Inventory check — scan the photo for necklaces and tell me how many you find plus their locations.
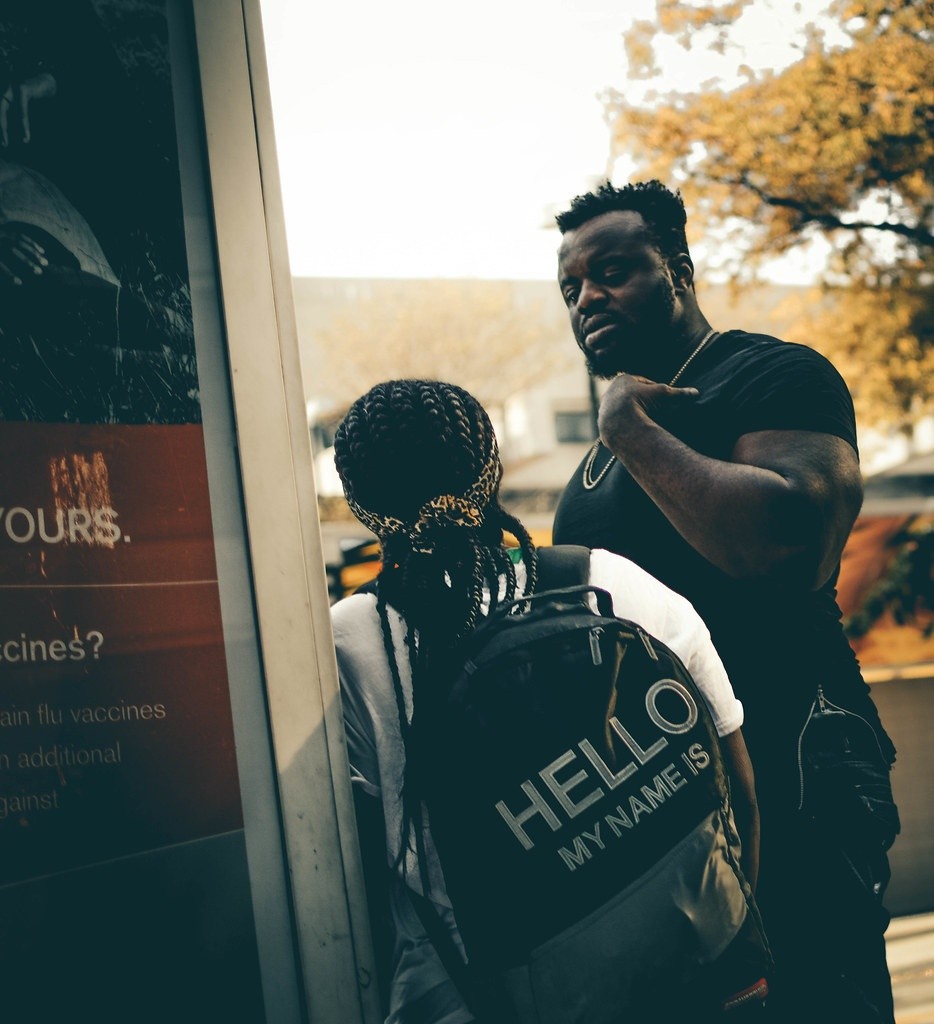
[582,329,721,490]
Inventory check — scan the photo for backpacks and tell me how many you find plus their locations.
[341,543,778,1023]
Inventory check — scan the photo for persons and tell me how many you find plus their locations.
[320,376,785,1024]
[538,179,902,1024]
